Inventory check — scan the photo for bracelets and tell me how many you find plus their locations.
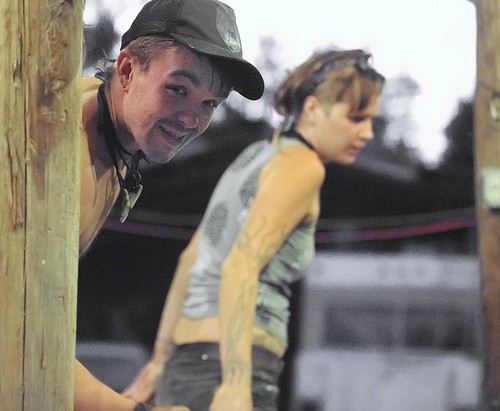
[133,402,152,410]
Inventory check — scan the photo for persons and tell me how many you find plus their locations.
[120,50,385,411]
[74,0,266,411]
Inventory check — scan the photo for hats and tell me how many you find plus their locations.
[120,0,265,100]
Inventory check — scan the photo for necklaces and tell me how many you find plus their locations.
[281,130,319,153]
[97,84,151,223]
